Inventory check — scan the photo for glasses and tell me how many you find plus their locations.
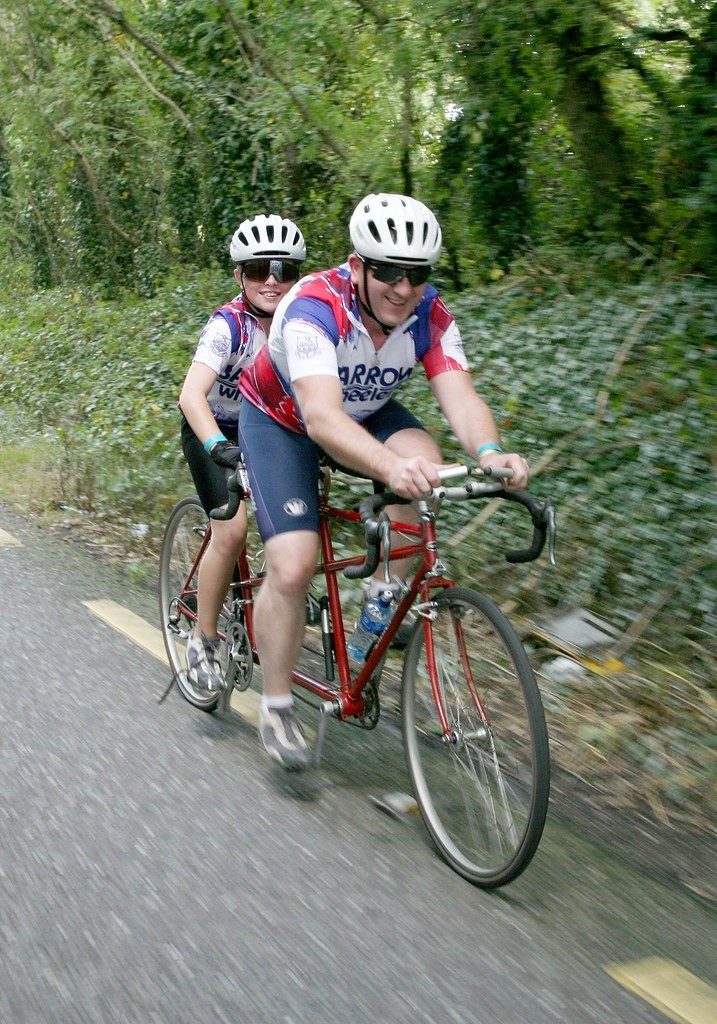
[355,252,431,287]
[241,260,299,284]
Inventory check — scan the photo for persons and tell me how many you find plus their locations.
[174,213,332,692]
[236,190,531,779]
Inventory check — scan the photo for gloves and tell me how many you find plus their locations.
[210,442,241,469]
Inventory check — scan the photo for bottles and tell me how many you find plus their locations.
[346,590,394,667]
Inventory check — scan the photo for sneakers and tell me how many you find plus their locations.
[258,702,315,772]
[359,585,422,646]
[183,629,225,693]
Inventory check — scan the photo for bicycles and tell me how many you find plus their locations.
[155,442,562,891]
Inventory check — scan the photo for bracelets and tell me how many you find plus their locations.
[477,442,505,456]
[478,448,502,468]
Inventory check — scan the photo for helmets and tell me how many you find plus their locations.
[348,194,442,267]
[230,213,306,263]
